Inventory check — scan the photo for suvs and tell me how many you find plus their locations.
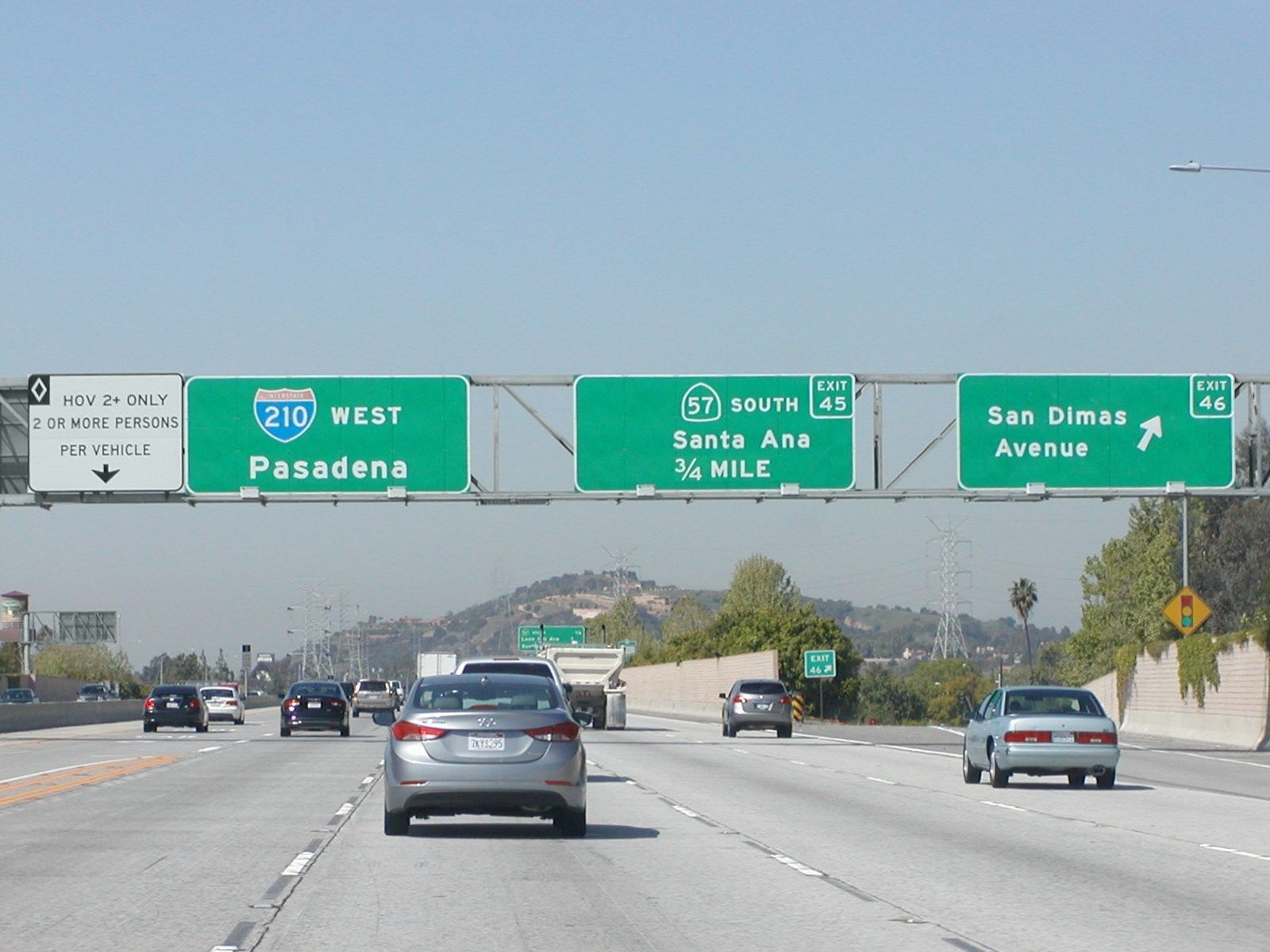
[719,680,792,739]
[350,679,395,719]
[452,657,593,729]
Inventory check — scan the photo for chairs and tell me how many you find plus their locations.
[296,689,309,695]
[511,694,538,709]
[432,695,463,710]
[324,688,335,695]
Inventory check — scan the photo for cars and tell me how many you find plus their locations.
[962,684,1123,791]
[275,681,352,739]
[371,674,590,842]
[77,684,115,702]
[0,688,39,704]
[142,686,210,735]
[200,687,246,724]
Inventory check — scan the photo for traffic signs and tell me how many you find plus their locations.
[803,650,837,678]
[572,375,856,494]
[517,626,585,652]
[956,369,1240,492]
[28,372,471,492]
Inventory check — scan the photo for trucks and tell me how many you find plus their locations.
[538,647,627,729]
